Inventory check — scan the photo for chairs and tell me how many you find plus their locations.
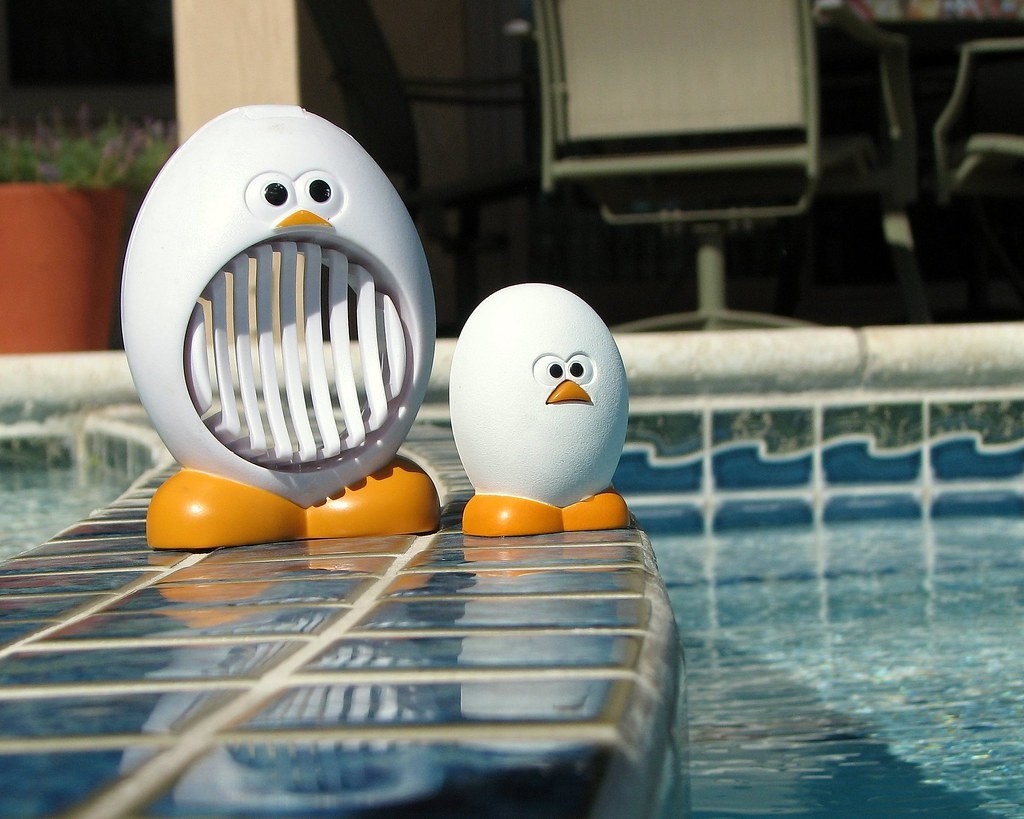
[531,1,1024,333]
[304,1,540,338]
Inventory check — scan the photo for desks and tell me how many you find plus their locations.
[814,19,1024,75]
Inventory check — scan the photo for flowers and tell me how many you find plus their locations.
[0,103,177,189]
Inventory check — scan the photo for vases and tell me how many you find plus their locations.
[0,181,125,353]
